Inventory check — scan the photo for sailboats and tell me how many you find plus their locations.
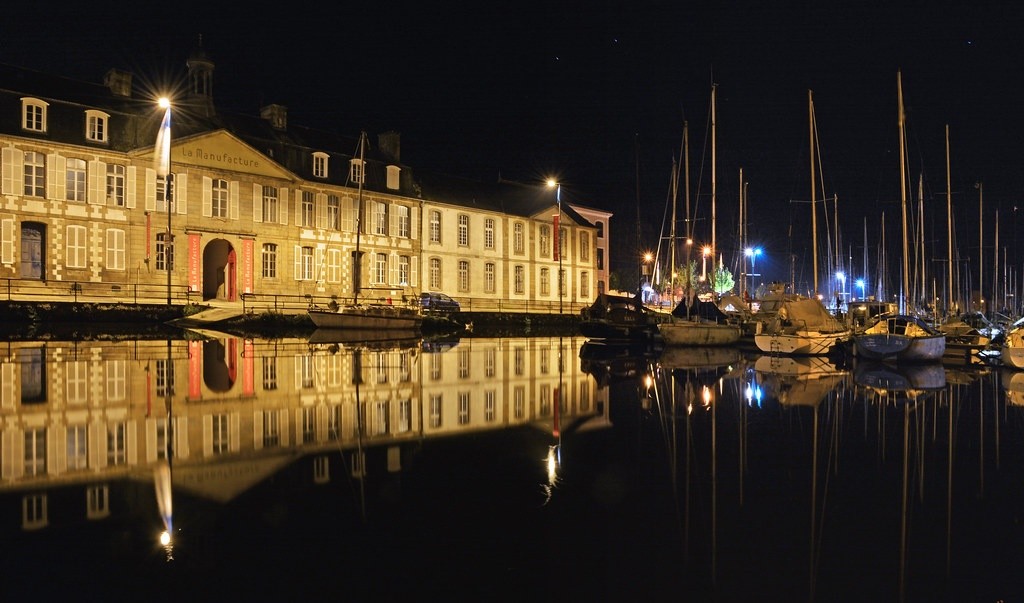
[659,71,1024,363]
[580,339,1024,602]
[308,328,422,522]
[306,131,458,342]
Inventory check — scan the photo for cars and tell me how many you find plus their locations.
[418,291,460,312]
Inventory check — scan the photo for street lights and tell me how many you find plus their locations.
[157,335,178,570]
[151,106,176,300]
[554,181,564,318]
[543,337,569,490]
